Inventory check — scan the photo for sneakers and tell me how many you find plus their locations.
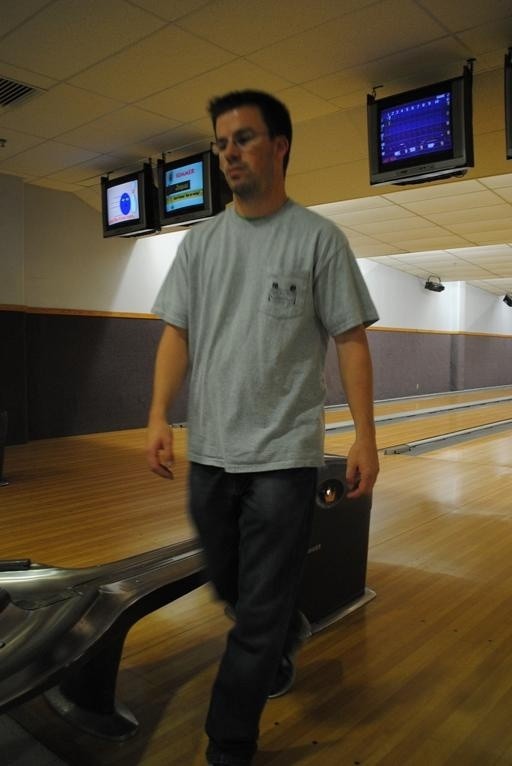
[268,611,311,699]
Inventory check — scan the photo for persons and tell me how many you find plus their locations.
[143,83,382,765]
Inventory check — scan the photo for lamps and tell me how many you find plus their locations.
[503,294,512,309]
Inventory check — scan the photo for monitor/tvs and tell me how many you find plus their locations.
[100,162,159,238]
[154,141,234,228]
[504,47,512,158]
[364,74,476,187]
